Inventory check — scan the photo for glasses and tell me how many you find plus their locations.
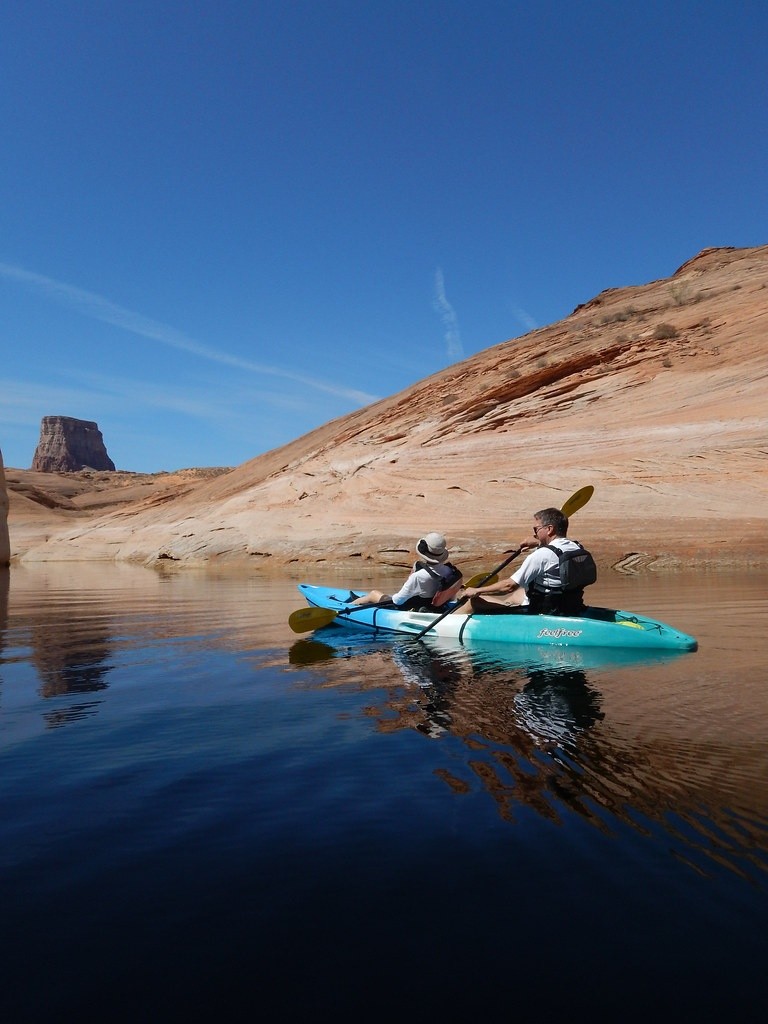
[533,524,550,536]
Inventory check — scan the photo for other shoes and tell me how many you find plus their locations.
[349,590,360,601]
[330,597,342,602]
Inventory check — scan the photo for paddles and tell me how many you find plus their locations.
[288,573,499,633]
[415,484,595,639]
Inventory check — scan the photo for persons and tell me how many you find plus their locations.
[452,507,583,618]
[329,532,455,612]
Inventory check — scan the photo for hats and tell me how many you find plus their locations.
[416,533,448,562]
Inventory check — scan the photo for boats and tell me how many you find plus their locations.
[297,584,698,650]
